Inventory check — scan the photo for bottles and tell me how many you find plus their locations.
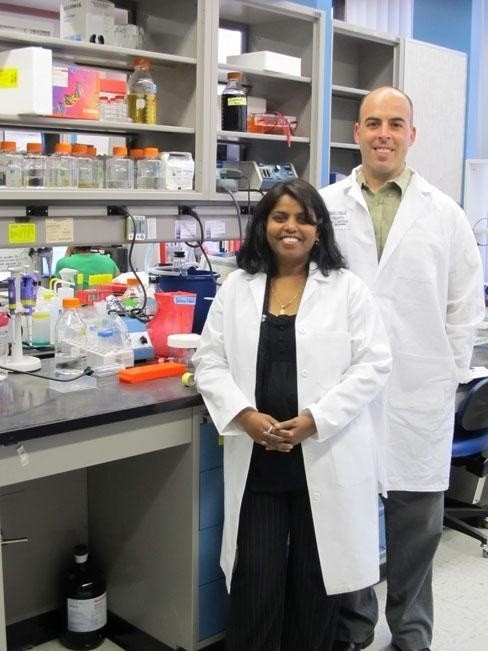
[0,140,162,189]
[32,312,51,346]
[123,57,157,127]
[76,273,116,300]
[54,298,86,375]
[0,313,10,382]
[59,545,109,651]
[121,280,146,317]
[172,252,187,277]
[221,72,247,134]
[88,327,112,355]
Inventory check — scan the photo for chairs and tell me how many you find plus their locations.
[442,379,488,557]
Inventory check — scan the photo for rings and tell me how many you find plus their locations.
[267,425,274,436]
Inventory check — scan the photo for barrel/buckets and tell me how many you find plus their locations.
[158,150,195,191]
[146,291,198,359]
[157,266,221,336]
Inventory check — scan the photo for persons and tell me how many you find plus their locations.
[55,246,121,290]
[316,87,485,651]
[193,180,390,651]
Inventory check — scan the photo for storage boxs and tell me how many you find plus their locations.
[227,48,302,79]
[114,8,146,50]
[49,60,129,121]
[1,46,53,116]
[57,1,116,46]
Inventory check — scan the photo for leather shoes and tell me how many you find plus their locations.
[331,631,373,650]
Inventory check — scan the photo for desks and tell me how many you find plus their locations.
[0,343,488,651]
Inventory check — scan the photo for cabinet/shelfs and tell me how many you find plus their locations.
[330,18,404,186]
[213,0,326,203]
[0,0,212,208]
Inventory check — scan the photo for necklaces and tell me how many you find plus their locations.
[267,275,305,317]
